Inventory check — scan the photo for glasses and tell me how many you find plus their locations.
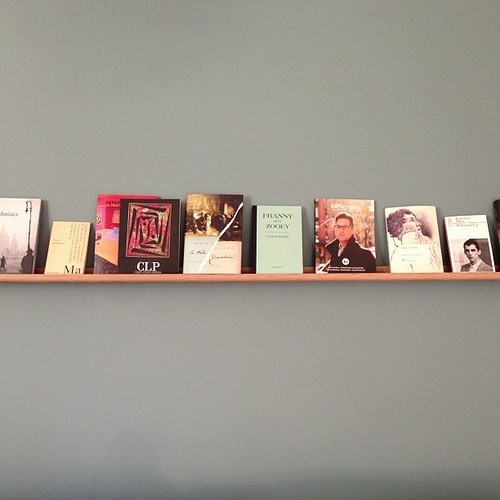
[334,224,351,230]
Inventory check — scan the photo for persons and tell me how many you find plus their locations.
[386,208,441,271]
[460,239,493,271]
[323,211,376,273]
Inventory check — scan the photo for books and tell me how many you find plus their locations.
[44,220,91,275]
[118,197,181,274]
[250,204,304,274]
[443,214,496,272]
[182,193,244,274]
[313,198,376,273]
[384,205,445,274]
[0,197,42,275]
[92,194,159,274]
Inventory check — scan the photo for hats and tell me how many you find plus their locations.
[26,249,34,252]
